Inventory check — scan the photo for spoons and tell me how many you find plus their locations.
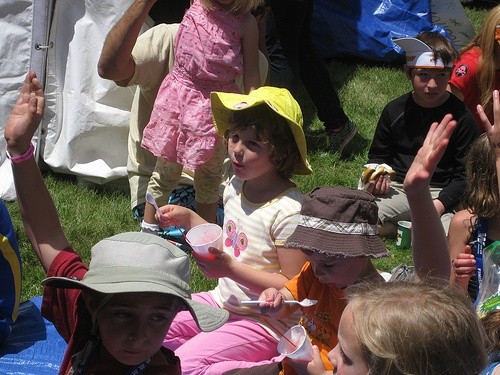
[240,298,318,307]
[146,192,168,221]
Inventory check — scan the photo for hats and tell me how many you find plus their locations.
[40,232,230,331]
[283,186,391,259]
[391,36,454,70]
[209,85,312,176]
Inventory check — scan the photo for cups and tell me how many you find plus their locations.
[276,325,314,362]
[395,220,411,250]
[185,223,223,262]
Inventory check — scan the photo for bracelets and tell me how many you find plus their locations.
[5,142,34,163]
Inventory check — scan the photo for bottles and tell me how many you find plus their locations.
[467,218,488,299]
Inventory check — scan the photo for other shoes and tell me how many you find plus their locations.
[326,122,368,164]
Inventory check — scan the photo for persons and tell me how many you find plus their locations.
[265,0,358,156]
[449,132,500,320]
[140,0,263,235]
[365,31,477,238]
[155,87,313,375]
[259,186,392,375]
[446,4,500,133]
[0,198,22,348]
[4,71,229,375]
[327,115,489,375]
[97,0,268,250]
